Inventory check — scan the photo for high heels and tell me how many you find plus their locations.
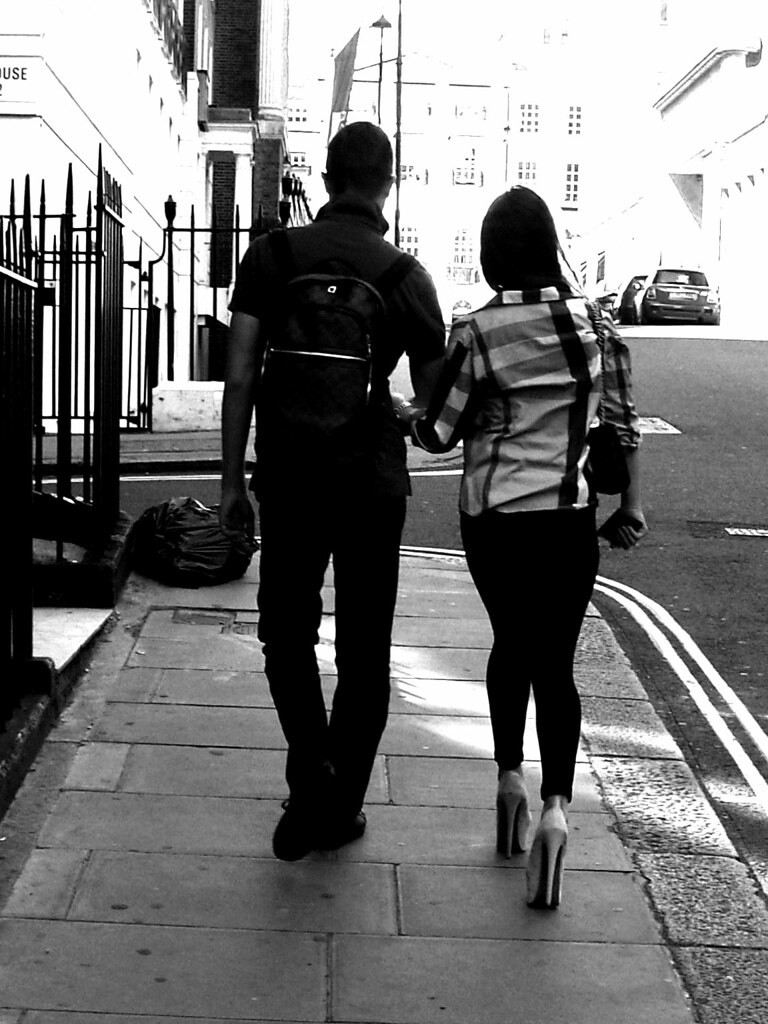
[496,772,529,860]
[524,808,569,910]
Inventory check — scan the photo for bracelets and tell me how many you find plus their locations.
[394,401,412,418]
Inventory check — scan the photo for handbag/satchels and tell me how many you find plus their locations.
[581,302,632,494]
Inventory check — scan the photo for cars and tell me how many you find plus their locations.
[619,269,720,325]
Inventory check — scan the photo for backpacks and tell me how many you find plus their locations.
[258,228,407,431]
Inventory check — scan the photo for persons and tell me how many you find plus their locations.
[217,123,448,861]
[388,184,647,908]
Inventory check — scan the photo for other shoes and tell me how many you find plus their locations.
[316,811,366,854]
[272,751,338,861]
